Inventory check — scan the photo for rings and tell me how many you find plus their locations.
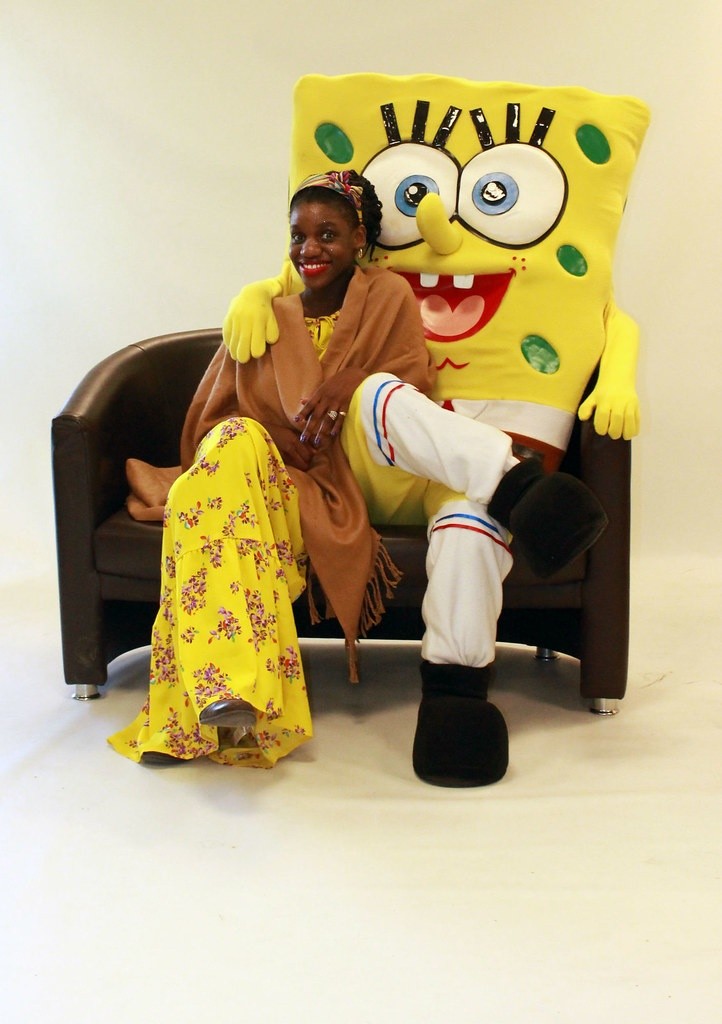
[339,411,346,416]
[326,411,338,420]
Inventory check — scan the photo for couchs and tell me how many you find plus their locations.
[51,328,632,715]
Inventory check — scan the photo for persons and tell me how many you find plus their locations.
[105,169,436,770]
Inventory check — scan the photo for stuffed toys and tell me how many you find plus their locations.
[221,73,651,789]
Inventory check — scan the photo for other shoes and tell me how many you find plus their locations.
[143,751,185,765]
[199,699,258,726]
